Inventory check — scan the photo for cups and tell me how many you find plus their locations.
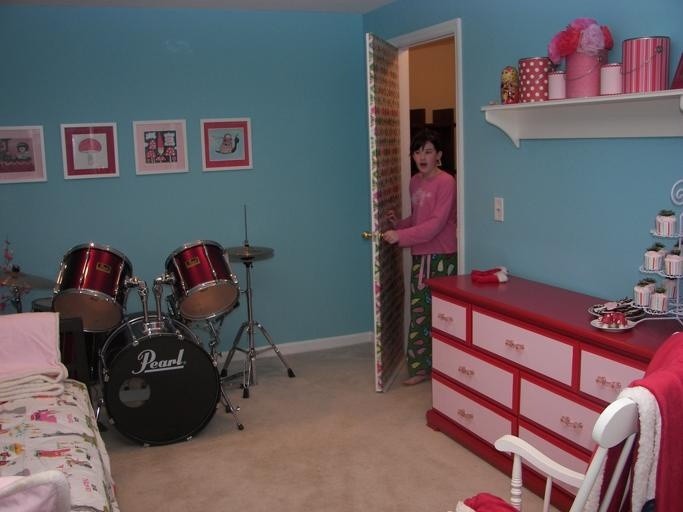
[548,70,567,101]
[599,62,623,97]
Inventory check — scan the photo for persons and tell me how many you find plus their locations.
[384,130,457,385]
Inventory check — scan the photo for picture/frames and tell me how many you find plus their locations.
[0,126,47,184]
[60,121,120,180]
[200,118,254,172]
[133,118,189,175]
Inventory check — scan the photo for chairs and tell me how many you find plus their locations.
[454,332,683,511]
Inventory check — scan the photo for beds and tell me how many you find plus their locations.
[0,366,113,512]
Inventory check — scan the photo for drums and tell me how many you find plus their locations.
[166,239,238,321]
[100,315,222,445]
[33,297,53,310]
[53,241,131,332]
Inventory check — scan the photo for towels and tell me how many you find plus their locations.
[584,385,662,512]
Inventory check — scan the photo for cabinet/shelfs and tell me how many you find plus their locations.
[423,272,682,511]
[589,179,683,333]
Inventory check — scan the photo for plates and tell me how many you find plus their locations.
[587,303,647,320]
[590,318,636,333]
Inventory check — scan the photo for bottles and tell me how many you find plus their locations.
[501,65,520,104]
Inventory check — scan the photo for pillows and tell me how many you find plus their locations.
[0,469,71,512]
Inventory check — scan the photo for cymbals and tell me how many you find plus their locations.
[226,246,273,261]
[2,270,57,290]
